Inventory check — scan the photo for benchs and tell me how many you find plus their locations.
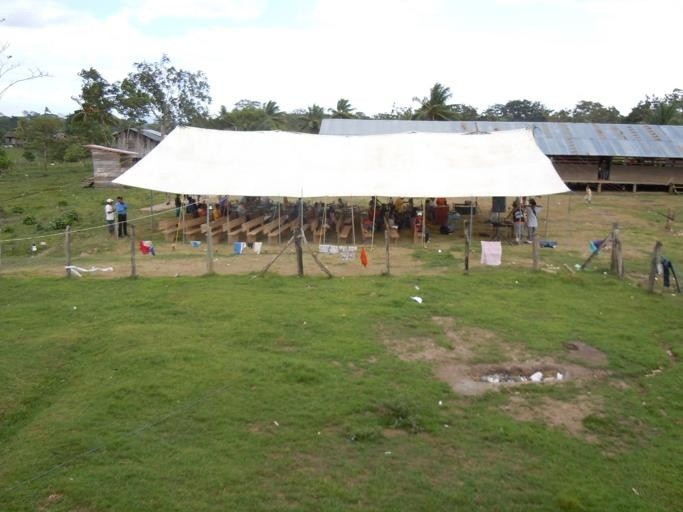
[157,210,423,245]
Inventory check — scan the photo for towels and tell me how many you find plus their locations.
[481,241,502,267]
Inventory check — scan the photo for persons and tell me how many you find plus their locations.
[114,196,128,238]
[584,184,592,206]
[174,194,461,237]
[504,195,543,243]
[104,198,116,233]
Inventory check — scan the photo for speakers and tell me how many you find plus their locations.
[492,197,506,213]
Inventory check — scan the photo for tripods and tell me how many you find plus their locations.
[489,212,513,245]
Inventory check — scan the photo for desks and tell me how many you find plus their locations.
[455,204,476,215]
[433,206,448,224]
